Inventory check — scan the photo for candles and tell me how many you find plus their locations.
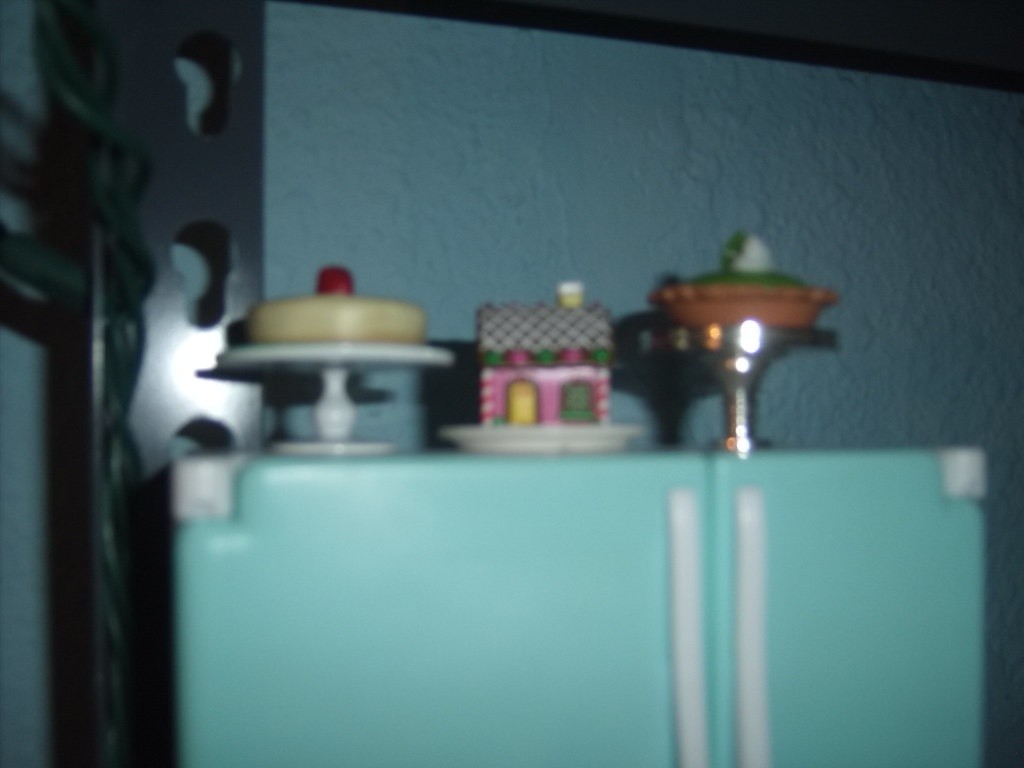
[669,488,713,768]
[737,488,774,768]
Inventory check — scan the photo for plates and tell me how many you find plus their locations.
[433,417,646,458]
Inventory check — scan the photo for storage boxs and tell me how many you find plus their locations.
[175,449,987,768]
